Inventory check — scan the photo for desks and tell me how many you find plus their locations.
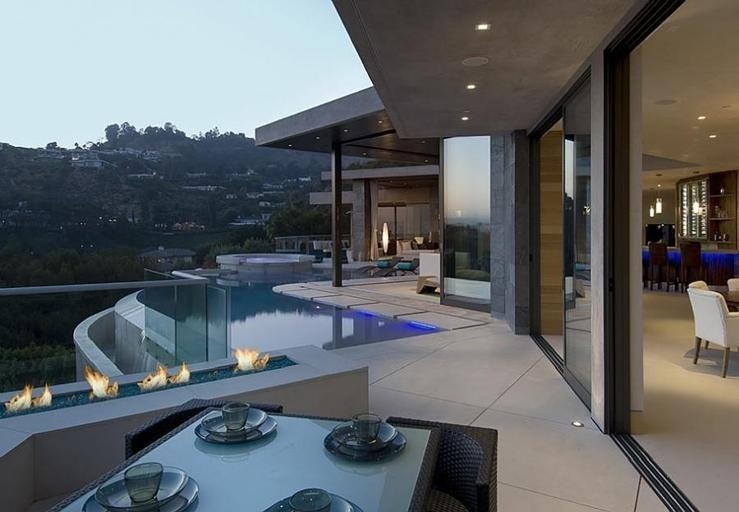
[642,248,739,286]
[45,406,445,511]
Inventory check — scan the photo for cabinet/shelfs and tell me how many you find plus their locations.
[707,169,739,243]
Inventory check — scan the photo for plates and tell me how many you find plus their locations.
[324,419,407,462]
[262,491,364,512]
[194,409,278,444]
[83,465,199,512]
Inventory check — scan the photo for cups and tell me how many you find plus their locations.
[352,411,381,445]
[124,462,163,505]
[221,401,250,432]
[288,488,333,512]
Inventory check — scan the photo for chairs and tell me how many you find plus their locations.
[685,277,739,377]
[648,242,679,292]
[386,412,499,511]
[680,241,704,295]
[122,396,284,458]
[306,236,440,293]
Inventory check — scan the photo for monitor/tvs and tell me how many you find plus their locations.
[646,224,676,246]
